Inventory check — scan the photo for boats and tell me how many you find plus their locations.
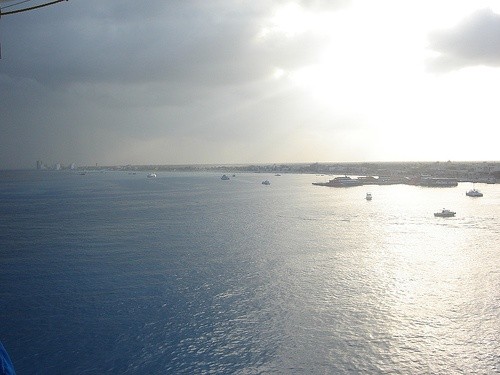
[465,189,484,198]
[426,174,498,189]
[221,174,229,181]
[432,207,456,217]
[147,173,157,178]
[366,193,373,200]
[261,180,270,184]
